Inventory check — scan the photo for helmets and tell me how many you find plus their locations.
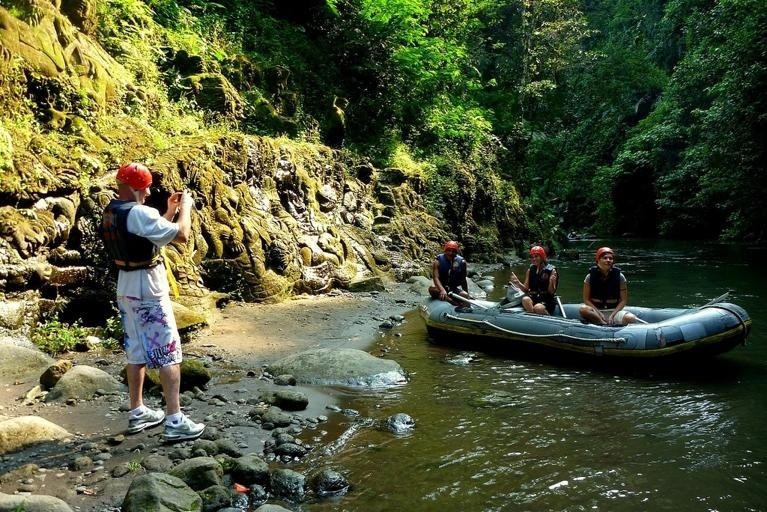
[596,247,614,261]
[445,241,458,251]
[531,246,546,260]
[116,163,153,191]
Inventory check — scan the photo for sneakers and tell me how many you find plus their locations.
[128,407,165,434]
[162,414,205,440]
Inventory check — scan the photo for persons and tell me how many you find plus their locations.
[102,162,207,442]
[427,240,477,306]
[509,245,559,316]
[567,229,575,239]
[578,247,641,326]
[568,232,580,240]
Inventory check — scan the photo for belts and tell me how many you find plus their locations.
[115,254,159,267]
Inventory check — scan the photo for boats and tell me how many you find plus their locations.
[414,293,754,369]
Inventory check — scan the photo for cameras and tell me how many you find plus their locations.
[177,193,183,202]
